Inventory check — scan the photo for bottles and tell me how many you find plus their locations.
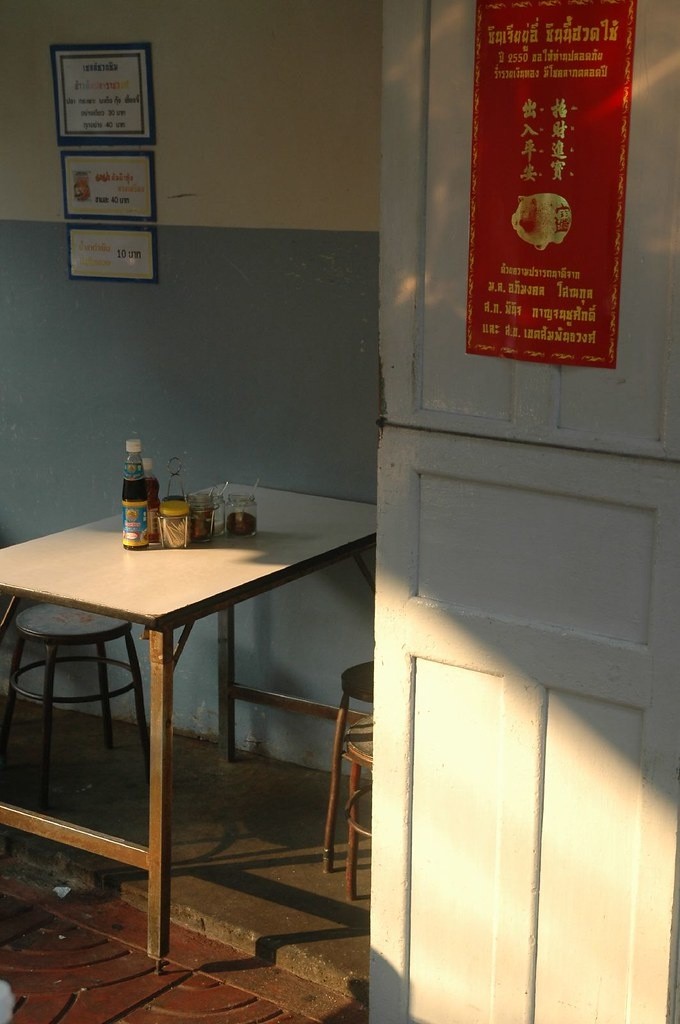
[225,493,257,538]
[158,500,191,548]
[141,458,161,545]
[211,493,226,537]
[186,493,215,542]
[121,438,150,550]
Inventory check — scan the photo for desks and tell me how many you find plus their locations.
[0,483,372,975]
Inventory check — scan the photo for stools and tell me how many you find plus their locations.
[323,660,373,900]
[0,603,149,811]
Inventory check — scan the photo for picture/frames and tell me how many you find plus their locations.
[49,43,158,148]
[66,223,159,284]
[60,150,158,223]
